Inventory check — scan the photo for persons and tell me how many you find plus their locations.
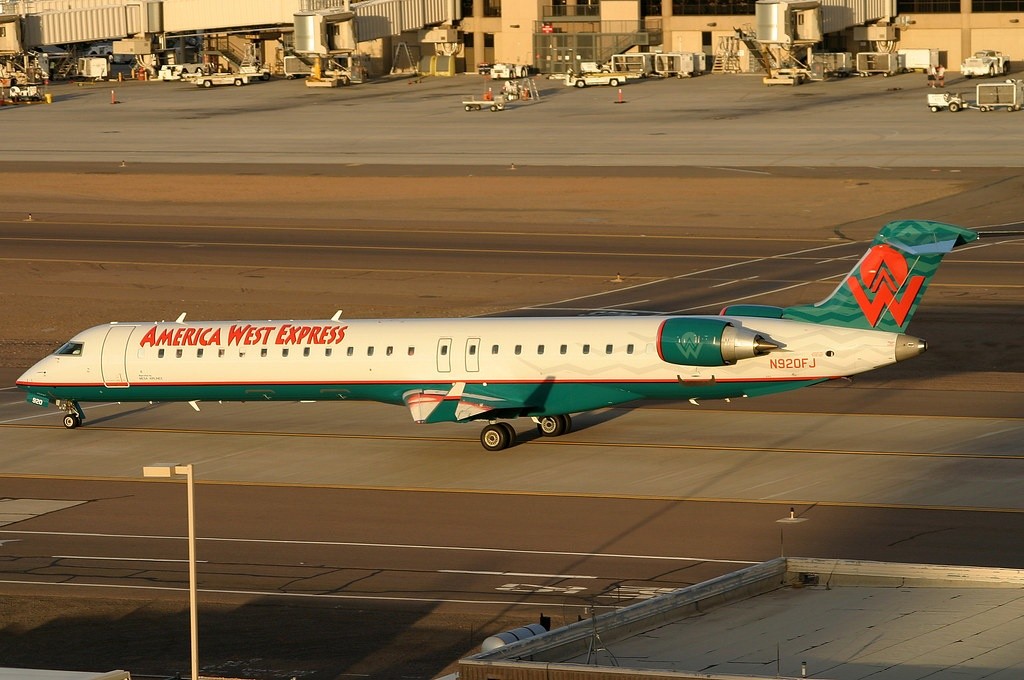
[926,63,946,88]
[49,60,56,81]
[72,346,80,355]
[568,68,586,82]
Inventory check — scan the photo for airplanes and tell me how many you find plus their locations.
[13,212,977,452]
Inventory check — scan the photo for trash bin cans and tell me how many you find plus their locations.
[478,63,490,75]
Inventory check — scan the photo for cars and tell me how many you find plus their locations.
[832,67,855,79]
[961,49,1011,80]
